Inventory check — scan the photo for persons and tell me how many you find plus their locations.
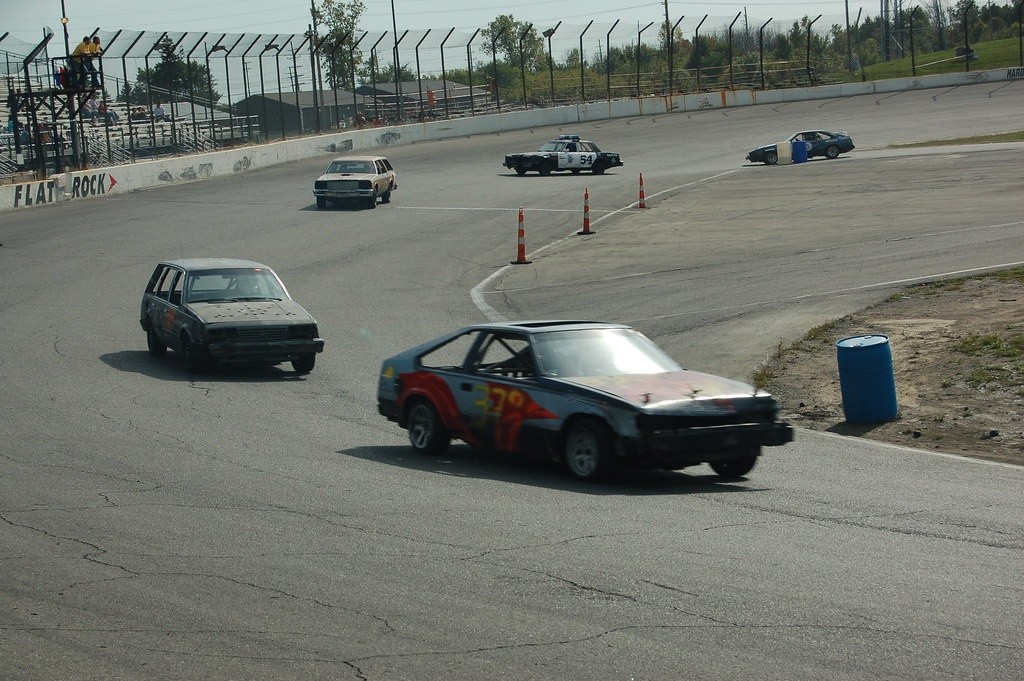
[7,113,54,143]
[67,35,104,89]
[132,101,170,122]
[82,90,120,127]
[228,270,262,295]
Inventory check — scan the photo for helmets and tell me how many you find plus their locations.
[236,273,259,293]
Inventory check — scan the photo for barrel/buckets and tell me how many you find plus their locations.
[793,141,807,162]
[836,334,898,423]
[777,142,792,164]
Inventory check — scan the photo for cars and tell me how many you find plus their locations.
[746,130,855,164]
[503,134,624,176]
[378,320,794,482]
[138,259,324,374]
[313,156,397,209]
